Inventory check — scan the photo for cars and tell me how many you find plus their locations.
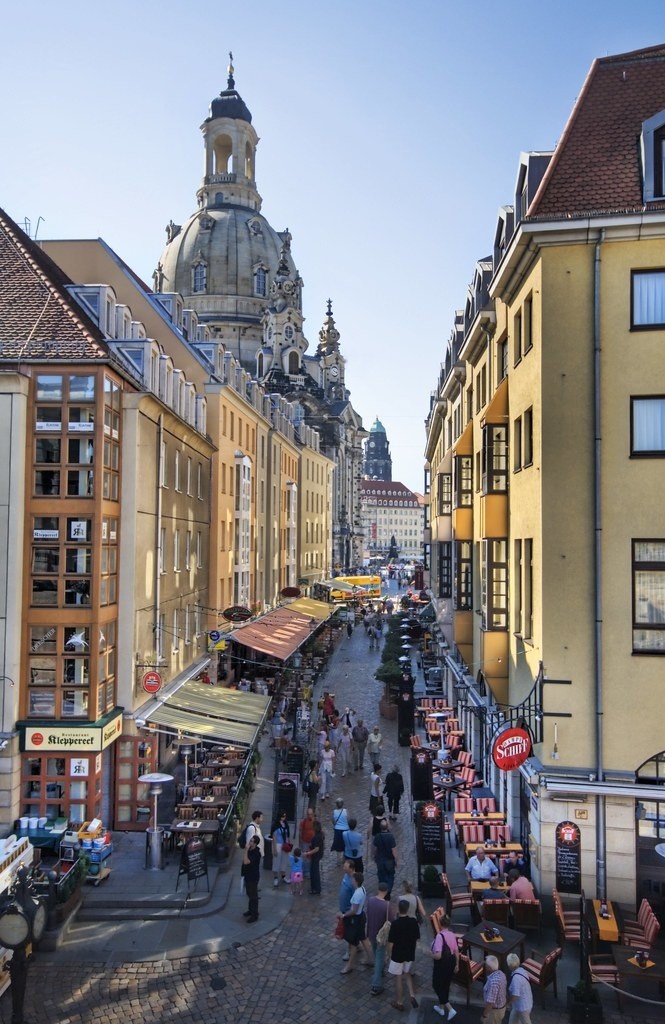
[332,612,363,623]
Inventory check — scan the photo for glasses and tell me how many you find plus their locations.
[281,815,286,818]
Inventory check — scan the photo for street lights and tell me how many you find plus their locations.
[1,864,50,1024]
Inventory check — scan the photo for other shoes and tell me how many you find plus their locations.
[389,815,397,822]
[308,889,320,894]
[340,966,353,974]
[410,996,418,1009]
[354,766,363,771]
[342,769,351,777]
[342,952,350,961]
[321,792,329,800]
[243,911,254,917]
[391,1000,404,1011]
[246,916,258,923]
[356,947,361,952]
[359,959,375,967]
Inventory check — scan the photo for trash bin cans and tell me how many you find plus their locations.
[408,608,415,617]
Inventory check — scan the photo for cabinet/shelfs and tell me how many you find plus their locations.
[59,839,112,886]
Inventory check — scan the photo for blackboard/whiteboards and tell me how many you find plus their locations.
[416,821,446,865]
[277,788,297,824]
[287,754,304,784]
[556,843,580,886]
[186,849,208,879]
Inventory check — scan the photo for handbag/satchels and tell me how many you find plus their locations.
[376,920,391,946]
[282,842,293,852]
[335,918,344,940]
[431,933,451,960]
[368,628,371,635]
[302,770,320,794]
[415,909,424,925]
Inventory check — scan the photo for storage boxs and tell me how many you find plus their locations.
[91,843,114,862]
[54,818,68,829]
[93,836,106,849]
[44,821,54,830]
[78,822,103,839]
[81,838,92,847]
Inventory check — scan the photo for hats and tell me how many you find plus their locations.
[508,869,519,877]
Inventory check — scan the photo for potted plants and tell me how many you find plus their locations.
[373,660,404,720]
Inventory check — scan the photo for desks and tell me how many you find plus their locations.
[585,899,625,947]
[468,880,536,899]
[453,812,505,825]
[168,746,247,855]
[421,742,452,759]
[432,758,465,774]
[8,828,71,854]
[471,788,496,799]
[460,920,526,972]
[464,841,524,863]
[433,774,467,812]
[612,945,665,1009]
[427,712,451,723]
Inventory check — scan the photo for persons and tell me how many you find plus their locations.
[240,557,536,1024]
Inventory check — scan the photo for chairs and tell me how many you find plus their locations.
[279,626,343,705]
[177,804,193,816]
[180,808,194,819]
[188,787,202,796]
[222,768,236,776]
[203,808,217,821]
[212,786,229,795]
[198,768,214,776]
[410,697,661,1010]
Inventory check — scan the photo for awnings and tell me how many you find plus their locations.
[320,577,364,596]
[226,607,325,662]
[280,596,341,621]
[139,680,272,749]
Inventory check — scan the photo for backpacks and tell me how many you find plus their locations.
[237,824,256,849]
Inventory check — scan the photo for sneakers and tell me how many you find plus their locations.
[274,879,278,887]
[282,877,291,884]
[434,1006,444,1015]
[447,1008,457,1020]
[370,986,384,995]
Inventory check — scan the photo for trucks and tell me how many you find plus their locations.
[331,575,381,601]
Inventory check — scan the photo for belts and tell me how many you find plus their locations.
[492,1005,505,1009]
